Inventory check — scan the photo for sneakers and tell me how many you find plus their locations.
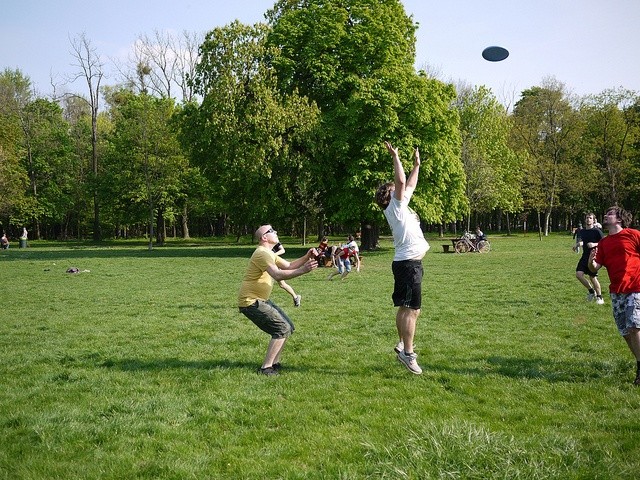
[272,363,282,369]
[588,290,597,301]
[394,342,416,354]
[398,350,422,374]
[596,297,604,304]
[294,295,301,307]
[258,367,278,375]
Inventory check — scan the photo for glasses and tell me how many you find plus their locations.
[350,248,354,250]
[260,228,273,239]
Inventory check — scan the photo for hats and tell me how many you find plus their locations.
[349,244,355,247]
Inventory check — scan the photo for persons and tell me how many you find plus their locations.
[316,237,330,268]
[1,233,10,250]
[238,224,319,376]
[374,141,431,376]
[594,217,603,230]
[328,245,360,281]
[345,235,360,267]
[460,230,476,240]
[272,242,301,307]
[572,223,582,253]
[20,227,28,240]
[474,227,487,251]
[573,211,605,304]
[588,204,640,386]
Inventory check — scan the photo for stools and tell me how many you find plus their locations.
[442,245,450,253]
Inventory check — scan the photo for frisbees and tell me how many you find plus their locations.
[482,46,509,62]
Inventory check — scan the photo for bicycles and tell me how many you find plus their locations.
[454,233,490,254]
